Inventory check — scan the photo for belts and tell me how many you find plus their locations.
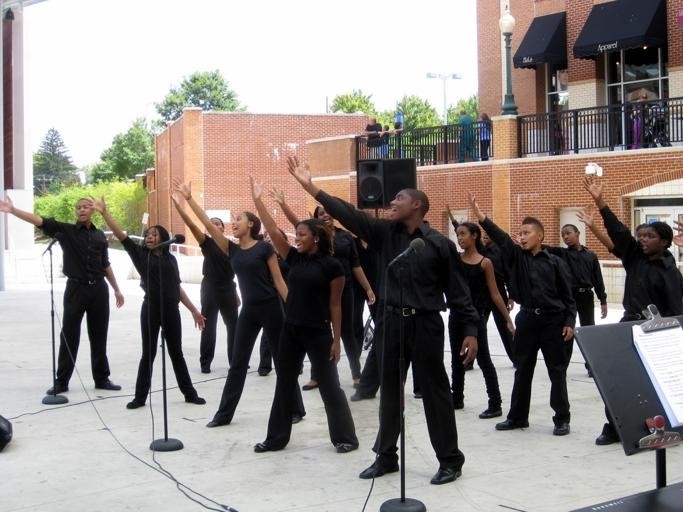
[523,306,559,315]
[384,304,423,317]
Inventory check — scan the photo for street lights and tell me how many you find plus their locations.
[498,3,520,115]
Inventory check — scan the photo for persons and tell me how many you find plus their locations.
[466,188,575,436]
[576,176,683,444]
[366,117,402,159]
[0,189,127,396]
[268,185,423,400]
[284,154,482,485]
[477,113,491,161]
[249,175,359,453]
[446,205,514,418]
[455,110,480,163]
[166,175,289,425]
[84,193,210,410]
[512,224,607,377]
[631,93,671,149]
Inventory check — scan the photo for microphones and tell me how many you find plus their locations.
[151,234,185,251]
[386,238,426,270]
[43,232,64,255]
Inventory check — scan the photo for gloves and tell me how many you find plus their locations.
[573,287,592,294]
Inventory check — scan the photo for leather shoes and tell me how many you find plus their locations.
[47,363,621,484]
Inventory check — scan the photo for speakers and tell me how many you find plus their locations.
[356,157,417,210]
